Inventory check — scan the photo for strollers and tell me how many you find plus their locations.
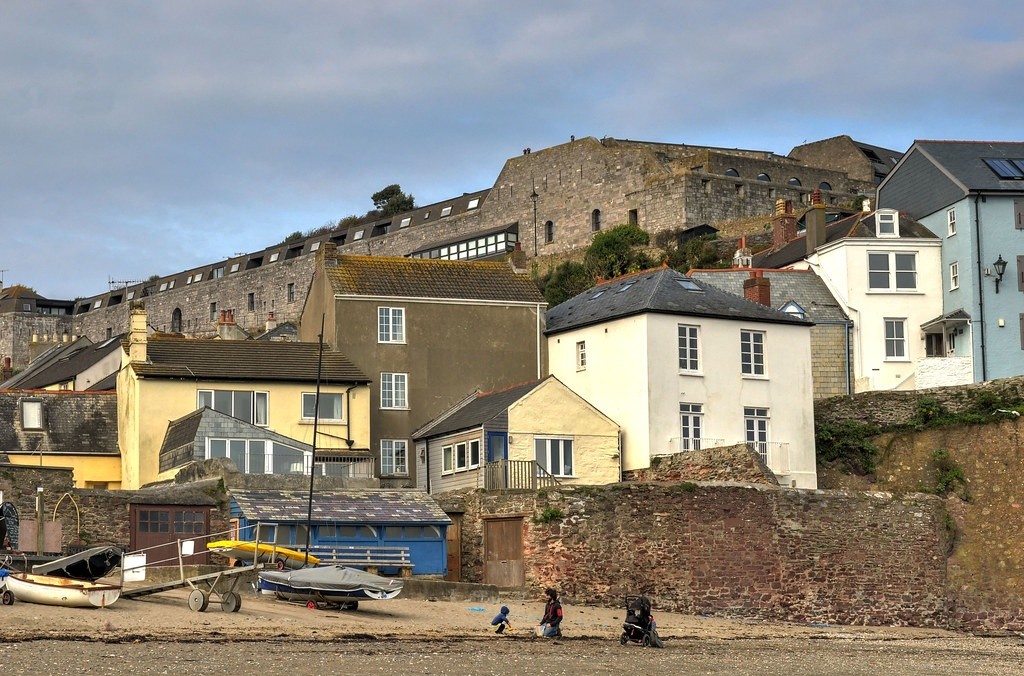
[621,594,658,646]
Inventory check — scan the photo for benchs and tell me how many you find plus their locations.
[276,544,415,579]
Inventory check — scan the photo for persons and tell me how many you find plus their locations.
[537,588,563,636]
[491,605,511,633]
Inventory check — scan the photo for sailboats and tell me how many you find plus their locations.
[253,312,405,603]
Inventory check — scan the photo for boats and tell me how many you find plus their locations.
[33,546,123,581]
[208,540,320,570]
[4,572,122,608]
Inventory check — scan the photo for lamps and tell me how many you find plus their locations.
[993,254,1008,293]
